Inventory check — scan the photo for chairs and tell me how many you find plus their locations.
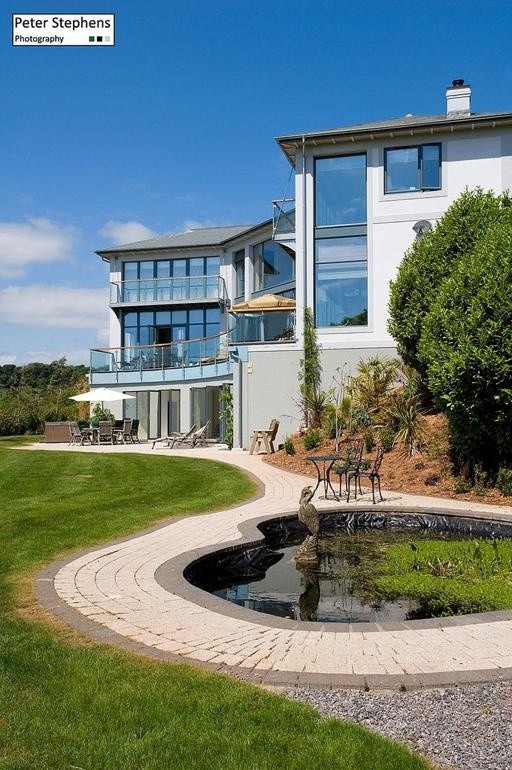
[328,441,386,503]
[151,418,212,450]
[249,419,280,456]
[67,418,141,446]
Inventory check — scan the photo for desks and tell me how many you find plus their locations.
[304,455,340,502]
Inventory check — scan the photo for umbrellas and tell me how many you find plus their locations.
[68,385,137,421]
[229,292,295,315]
